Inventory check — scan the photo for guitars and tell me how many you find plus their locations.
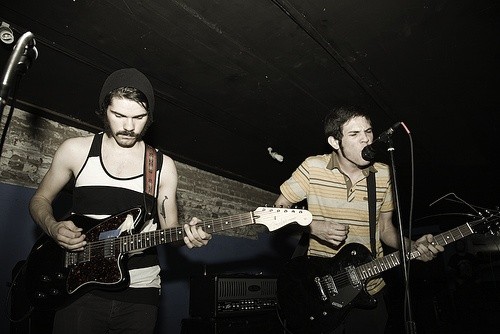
[24,203,313,311]
[276,205,500,334]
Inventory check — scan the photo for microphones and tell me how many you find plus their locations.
[361,122,403,161]
[0,31,38,120]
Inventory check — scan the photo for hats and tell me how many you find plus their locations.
[98,68,154,114]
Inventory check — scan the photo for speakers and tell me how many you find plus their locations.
[181,315,280,334]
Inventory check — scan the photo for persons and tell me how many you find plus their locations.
[29,68,211,334]
[272,103,445,334]
